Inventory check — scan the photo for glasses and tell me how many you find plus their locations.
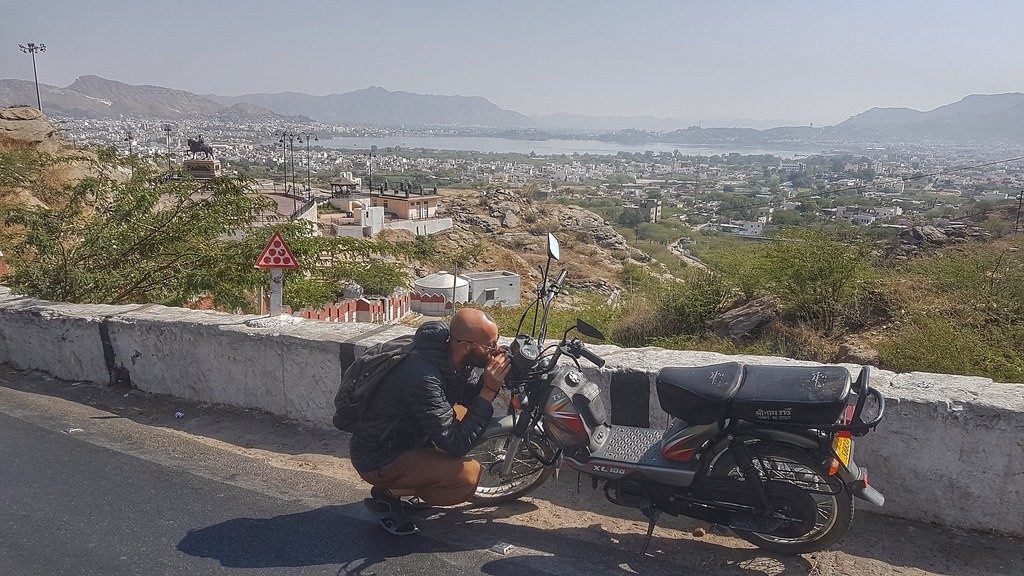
[457,339,497,354]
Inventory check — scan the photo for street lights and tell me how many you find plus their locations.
[274,129,293,193]
[363,149,376,195]
[124,130,134,175]
[298,132,319,200]
[280,134,303,213]
[18,42,47,111]
[163,123,173,170]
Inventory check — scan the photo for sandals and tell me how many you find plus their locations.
[364,486,418,535]
[399,493,431,510]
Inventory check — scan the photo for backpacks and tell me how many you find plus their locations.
[334,334,449,420]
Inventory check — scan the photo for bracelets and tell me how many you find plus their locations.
[483,384,496,393]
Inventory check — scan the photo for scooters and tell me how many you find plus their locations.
[461,232,887,560]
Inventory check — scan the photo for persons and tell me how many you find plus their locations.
[197,134,204,146]
[349,308,511,535]
